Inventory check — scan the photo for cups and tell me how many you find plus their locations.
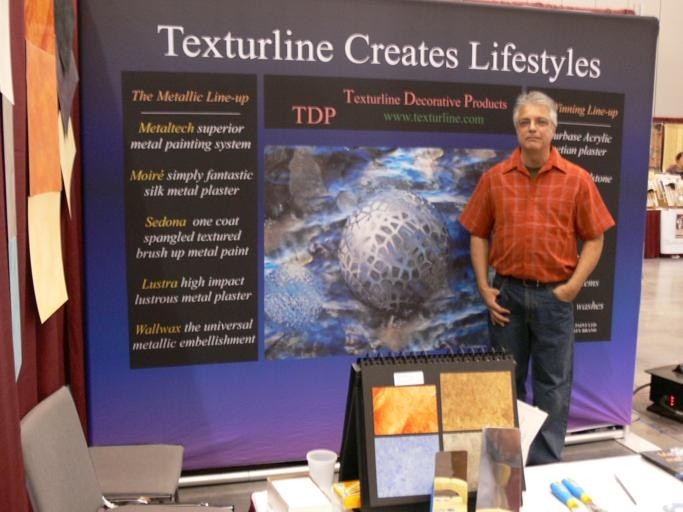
[306,448,338,492]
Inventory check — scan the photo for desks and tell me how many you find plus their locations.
[248,457,683,512]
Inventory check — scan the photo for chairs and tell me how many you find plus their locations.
[20,385,234,512]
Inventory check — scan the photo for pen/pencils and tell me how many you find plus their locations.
[613,472,638,506]
[550,480,607,512]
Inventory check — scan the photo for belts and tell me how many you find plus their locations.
[513,278,567,289]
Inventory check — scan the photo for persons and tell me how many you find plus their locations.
[456,91,618,468]
[666,154,682,178]
